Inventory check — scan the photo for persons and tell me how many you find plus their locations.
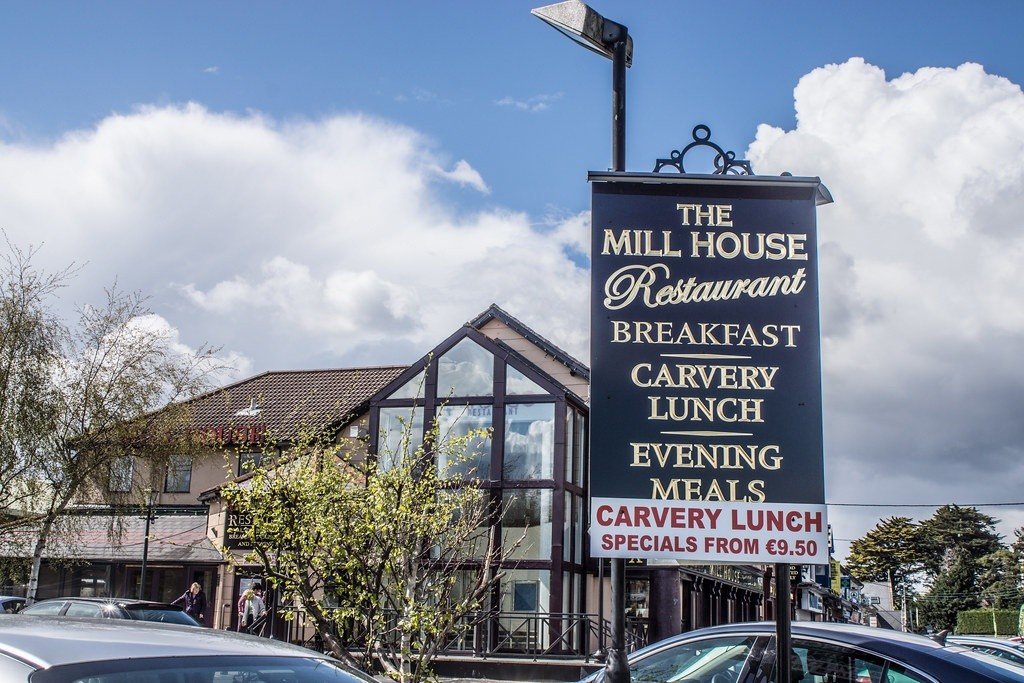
[171,582,207,623]
[236,582,268,633]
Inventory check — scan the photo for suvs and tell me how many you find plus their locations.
[15,596,202,627]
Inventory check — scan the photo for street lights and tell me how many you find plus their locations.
[528,0,636,683]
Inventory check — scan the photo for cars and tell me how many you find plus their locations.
[0,596,39,614]
[577,622,1024,683]
[0,614,383,683]
